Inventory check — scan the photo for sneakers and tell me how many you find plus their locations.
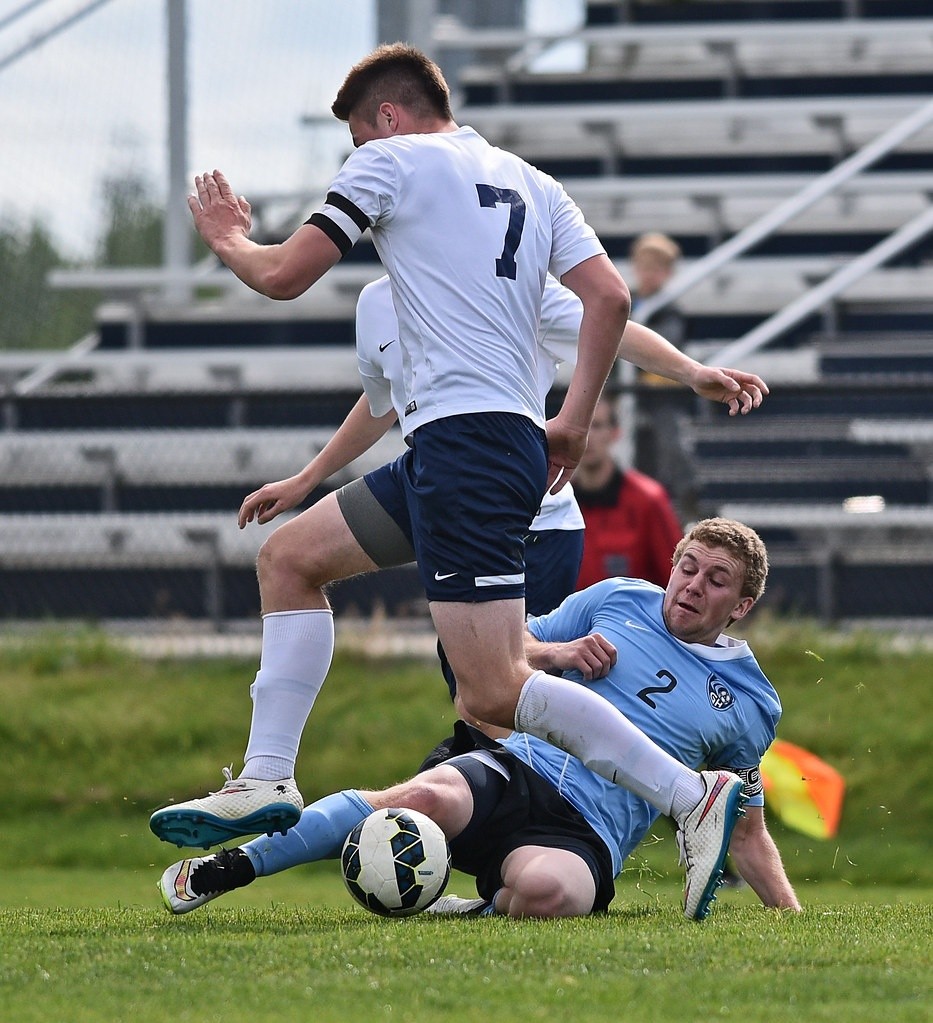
[156,847,243,913]
[674,768,752,920]
[147,762,304,850]
[426,893,488,916]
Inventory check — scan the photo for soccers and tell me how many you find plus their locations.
[340,806,452,917]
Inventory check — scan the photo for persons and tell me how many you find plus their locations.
[150,43,769,852]
[157,518,802,921]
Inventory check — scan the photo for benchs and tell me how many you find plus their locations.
[0,19,933,634]
